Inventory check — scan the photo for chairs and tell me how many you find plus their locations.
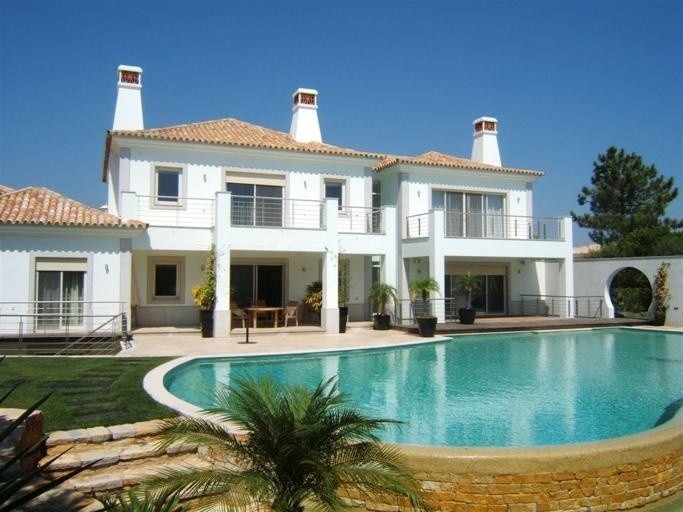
[230,299,299,329]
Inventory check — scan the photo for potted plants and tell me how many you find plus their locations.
[409,274,440,337]
[652,262,670,326]
[191,282,214,338]
[454,271,485,324]
[339,239,353,333]
[366,281,399,330]
[302,281,322,324]
